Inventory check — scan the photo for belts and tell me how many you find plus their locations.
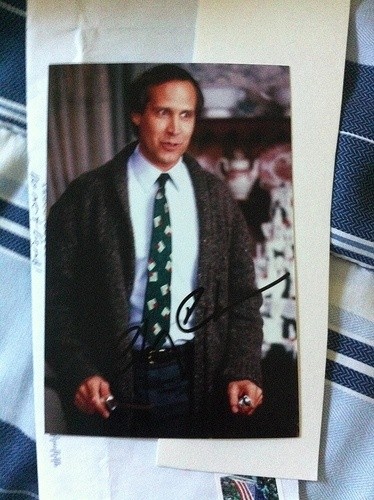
[128,339,196,369]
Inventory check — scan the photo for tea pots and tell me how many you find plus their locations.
[217,150,261,200]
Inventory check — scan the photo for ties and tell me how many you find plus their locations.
[142,173,173,367]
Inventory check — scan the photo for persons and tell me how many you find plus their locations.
[223,133,275,258]
[45,64,264,439]
